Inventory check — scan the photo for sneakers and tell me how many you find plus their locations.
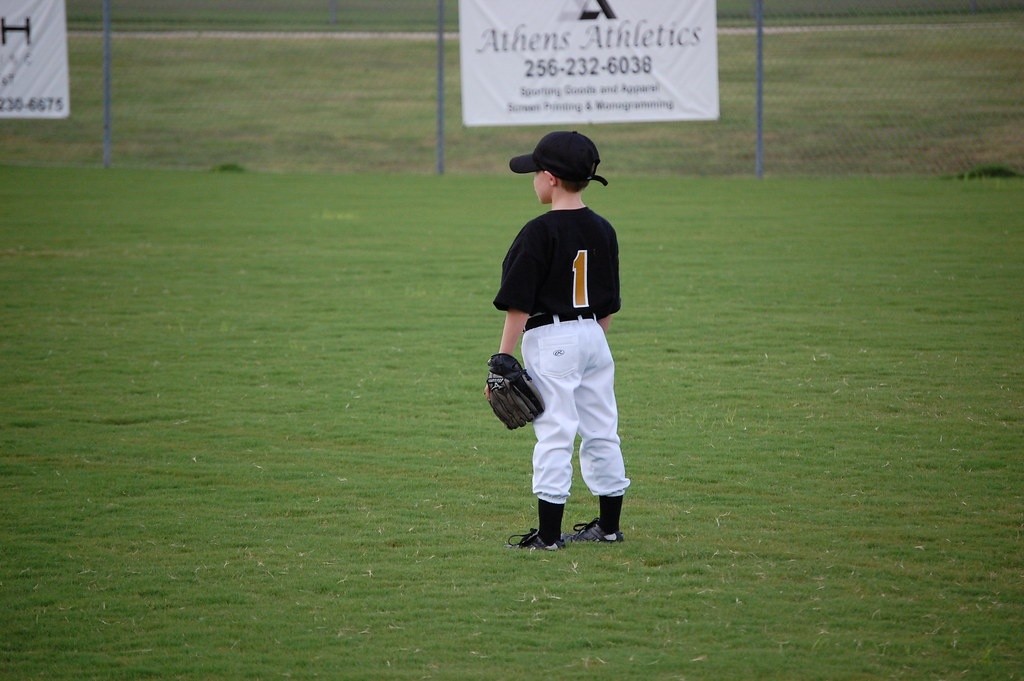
[507,533,566,551]
[563,521,624,542]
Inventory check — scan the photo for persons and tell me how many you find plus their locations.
[484,130,631,551]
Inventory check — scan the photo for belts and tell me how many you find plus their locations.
[523,313,594,331]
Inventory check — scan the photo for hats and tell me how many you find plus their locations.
[509,130,609,186]
